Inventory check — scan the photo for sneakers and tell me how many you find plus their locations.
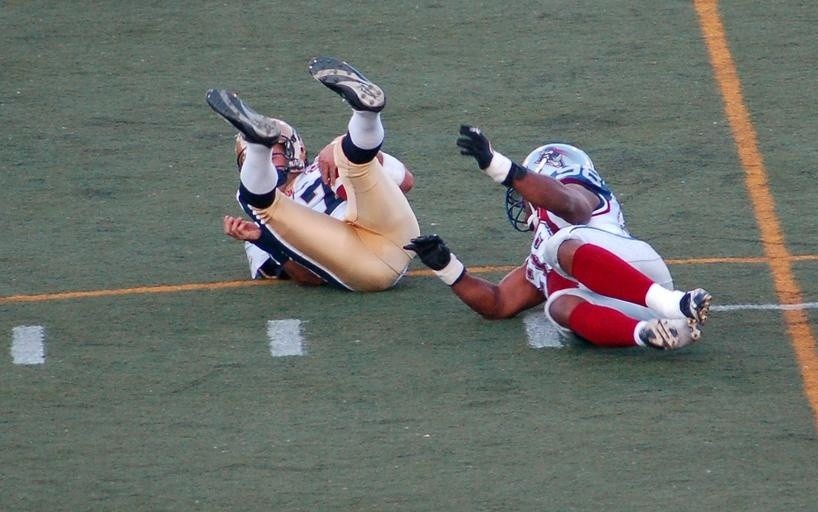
[204,87,282,150]
[636,316,702,352]
[306,55,387,114]
[679,287,713,326]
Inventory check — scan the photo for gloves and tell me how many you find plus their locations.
[454,121,517,189]
[402,232,466,289]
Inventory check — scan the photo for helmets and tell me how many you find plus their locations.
[506,143,595,232]
[233,115,307,175]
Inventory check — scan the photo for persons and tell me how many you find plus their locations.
[205,54,421,293]
[402,121,715,354]
